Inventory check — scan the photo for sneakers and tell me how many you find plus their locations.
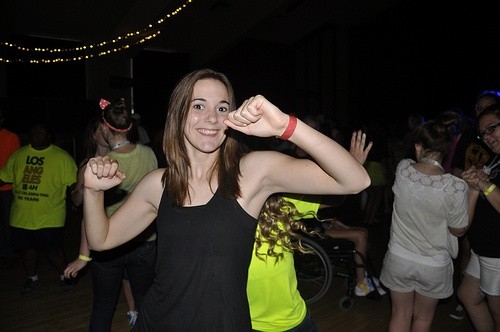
[354,275,388,298]
[449,302,467,320]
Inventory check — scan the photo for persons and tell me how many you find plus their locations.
[310,211,382,297]
[94,96,161,327]
[53,125,80,165]
[0,127,22,257]
[239,129,374,331]
[71,117,142,329]
[2,119,81,296]
[379,111,487,332]
[443,91,500,184]
[454,102,500,332]
[79,68,373,331]
[266,108,391,212]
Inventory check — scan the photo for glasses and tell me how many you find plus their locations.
[477,123,500,140]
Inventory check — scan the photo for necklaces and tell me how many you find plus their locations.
[420,157,445,173]
[110,141,132,150]
[483,154,500,175]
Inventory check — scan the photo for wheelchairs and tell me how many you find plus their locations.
[289,195,387,314]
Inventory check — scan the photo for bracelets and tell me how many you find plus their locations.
[79,254,93,262]
[483,183,496,196]
[275,113,297,140]
